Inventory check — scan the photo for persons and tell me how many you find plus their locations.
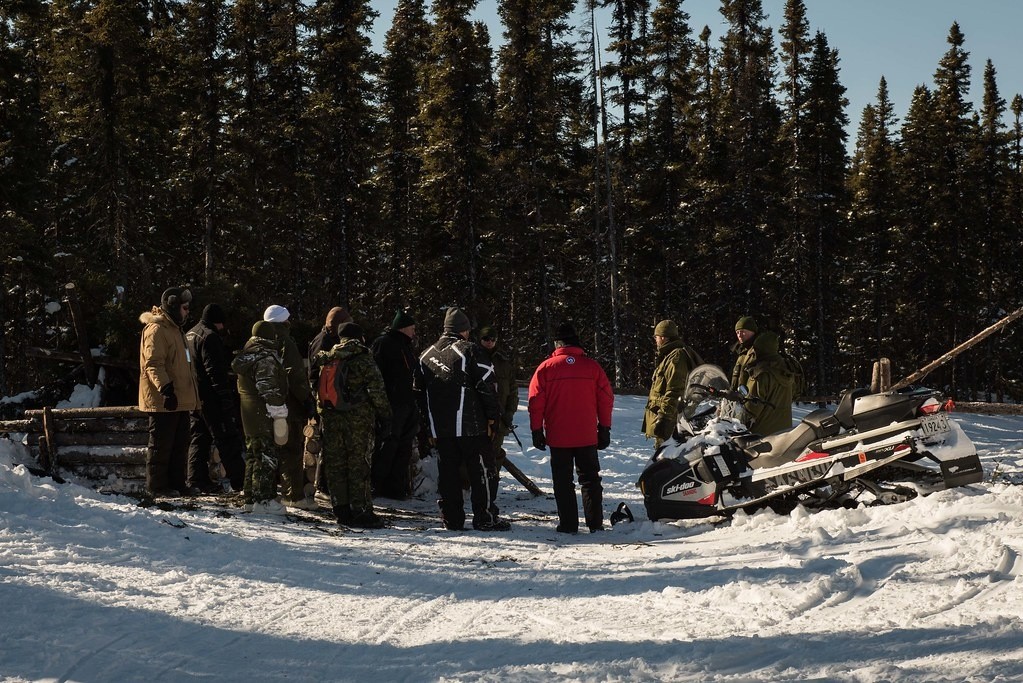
[418,306,511,531]
[311,322,390,528]
[307,306,352,384]
[477,324,519,514]
[138,286,202,497]
[737,332,796,437]
[231,304,318,510]
[641,319,704,450]
[730,315,759,393]
[185,303,246,493]
[370,309,418,497]
[528,325,614,534]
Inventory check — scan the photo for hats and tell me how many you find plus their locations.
[263,305,290,324]
[443,307,471,334]
[337,322,363,340]
[325,305,352,335]
[655,319,678,338]
[479,327,497,338]
[203,303,224,323]
[553,323,576,341]
[161,286,192,323]
[393,311,415,330]
[734,317,757,334]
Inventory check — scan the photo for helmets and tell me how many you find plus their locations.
[253,321,276,341]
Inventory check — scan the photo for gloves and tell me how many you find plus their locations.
[160,382,178,412]
[532,429,546,452]
[653,414,674,441]
[596,422,610,450]
[273,417,288,446]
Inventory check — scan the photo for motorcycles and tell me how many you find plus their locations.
[638,383,984,522]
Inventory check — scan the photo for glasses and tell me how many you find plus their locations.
[180,305,190,311]
[482,337,496,343]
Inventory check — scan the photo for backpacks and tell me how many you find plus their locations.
[315,357,367,417]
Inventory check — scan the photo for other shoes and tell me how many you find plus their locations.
[153,480,603,533]
